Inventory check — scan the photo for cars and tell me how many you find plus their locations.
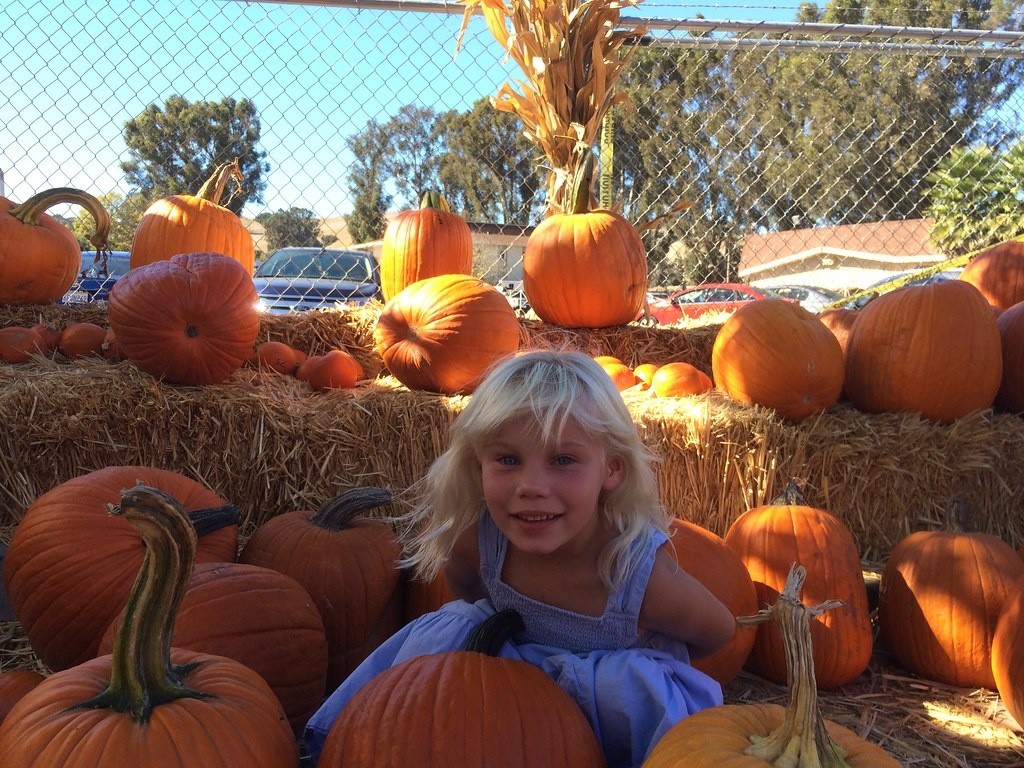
[635,282,798,326]
[251,247,383,313]
[504,289,531,312]
[647,293,667,302]
[755,285,855,313]
[58,250,132,306]
[846,269,966,309]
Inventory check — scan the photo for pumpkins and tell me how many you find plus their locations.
[0,159,1024,768]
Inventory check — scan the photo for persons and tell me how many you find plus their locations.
[303,349,734,768]
